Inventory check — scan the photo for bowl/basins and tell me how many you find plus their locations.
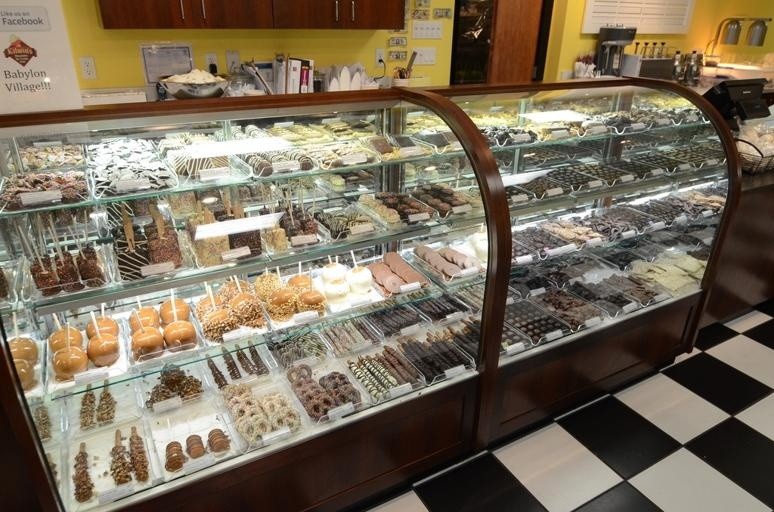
[156,77,230,99]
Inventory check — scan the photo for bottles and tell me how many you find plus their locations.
[690,51,697,72]
[673,50,680,66]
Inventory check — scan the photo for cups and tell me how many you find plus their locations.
[393,79,409,86]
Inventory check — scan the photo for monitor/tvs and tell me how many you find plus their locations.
[702,78,771,122]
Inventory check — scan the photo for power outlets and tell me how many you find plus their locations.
[376,48,385,67]
[81,55,96,79]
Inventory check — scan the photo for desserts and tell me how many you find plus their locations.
[0,89,731,503]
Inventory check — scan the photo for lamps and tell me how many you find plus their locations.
[704,16,772,66]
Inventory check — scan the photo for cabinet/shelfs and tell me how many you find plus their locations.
[272,0,406,30]
[0,87,501,511]
[95,1,273,29]
[403,73,743,451]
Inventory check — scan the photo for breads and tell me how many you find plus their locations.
[737,127,773,171]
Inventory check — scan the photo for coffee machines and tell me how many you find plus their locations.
[596,24,637,79]
